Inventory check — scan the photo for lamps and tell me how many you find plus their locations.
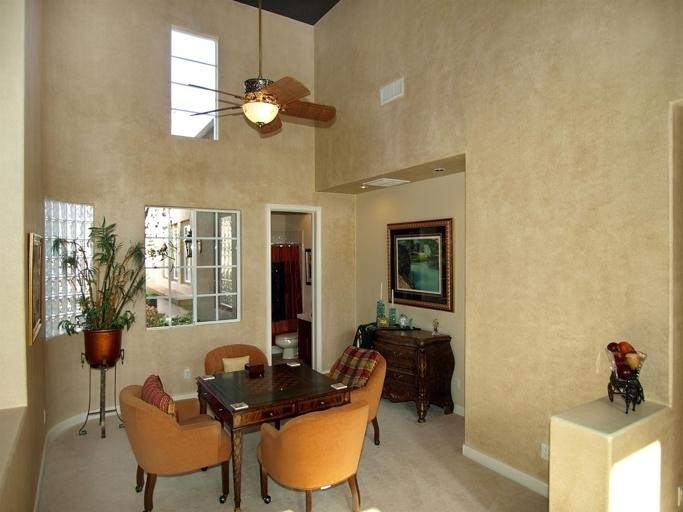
[184,230,202,257]
[242,97,279,127]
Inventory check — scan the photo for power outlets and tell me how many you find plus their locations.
[540,443,549,460]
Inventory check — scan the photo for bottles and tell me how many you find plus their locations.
[398,314,407,329]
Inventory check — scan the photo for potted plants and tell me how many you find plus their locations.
[51,217,177,366]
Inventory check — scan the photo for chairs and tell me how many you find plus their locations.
[256,400,369,512]
[205,344,268,373]
[323,354,386,446]
[118,373,232,512]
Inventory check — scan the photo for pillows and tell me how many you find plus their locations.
[222,355,250,372]
[142,375,180,424]
[328,345,381,389]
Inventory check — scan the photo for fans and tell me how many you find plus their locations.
[187,0,336,136]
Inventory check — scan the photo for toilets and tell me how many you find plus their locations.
[274,331,299,360]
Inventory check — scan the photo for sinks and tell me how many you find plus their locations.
[296,312,311,322]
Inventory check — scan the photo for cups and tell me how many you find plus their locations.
[244,362,264,379]
[377,317,389,329]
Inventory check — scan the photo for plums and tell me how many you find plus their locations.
[608,341,633,380]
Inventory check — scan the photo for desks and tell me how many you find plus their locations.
[195,362,350,512]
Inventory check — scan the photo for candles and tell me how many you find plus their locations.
[380,281,383,300]
[391,289,395,308]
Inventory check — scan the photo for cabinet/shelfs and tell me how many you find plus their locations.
[298,319,311,364]
[353,322,455,423]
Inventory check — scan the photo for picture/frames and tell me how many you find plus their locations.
[305,248,311,285]
[386,218,453,312]
[28,232,43,347]
